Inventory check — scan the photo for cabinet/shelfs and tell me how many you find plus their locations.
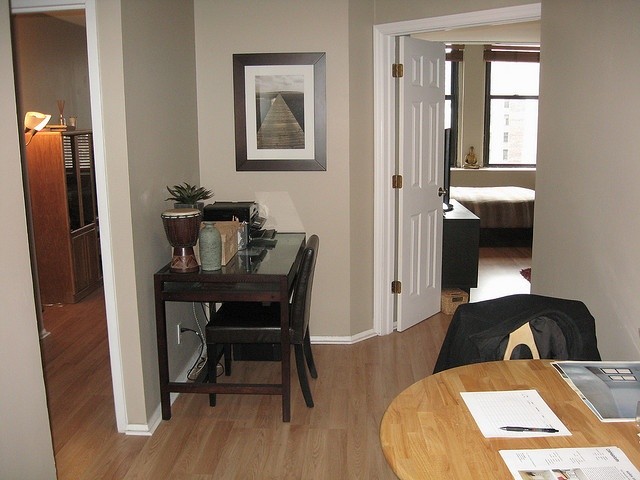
[444,198,481,298]
[24,129,102,305]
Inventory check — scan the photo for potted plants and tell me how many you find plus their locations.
[163,180,217,212]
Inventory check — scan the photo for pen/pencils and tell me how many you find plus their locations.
[499,426,559,433]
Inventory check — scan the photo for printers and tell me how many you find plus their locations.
[203,201,276,246]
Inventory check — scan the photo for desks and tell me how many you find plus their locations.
[153,233,306,422]
[378,359,640,480]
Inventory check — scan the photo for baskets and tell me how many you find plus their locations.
[441,288,469,315]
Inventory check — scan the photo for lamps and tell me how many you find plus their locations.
[23,111,51,147]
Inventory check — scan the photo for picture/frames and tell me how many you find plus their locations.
[232,52,325,171]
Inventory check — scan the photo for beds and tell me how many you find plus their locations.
[448,186,536,229]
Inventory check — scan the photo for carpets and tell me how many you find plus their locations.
[521,268,531,283]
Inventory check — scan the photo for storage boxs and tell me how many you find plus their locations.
[193,220,238,266]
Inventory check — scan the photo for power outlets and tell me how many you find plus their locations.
[175,322,190,346]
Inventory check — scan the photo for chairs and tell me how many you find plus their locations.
[207,236,319,406]
[434,293,602,373]
